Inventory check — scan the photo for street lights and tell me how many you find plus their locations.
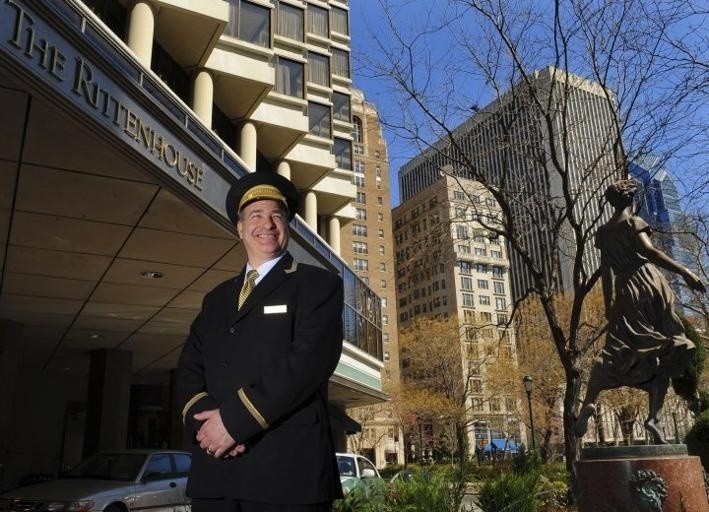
[523,374,535,449]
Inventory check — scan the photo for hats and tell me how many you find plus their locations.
[224,170,301,226]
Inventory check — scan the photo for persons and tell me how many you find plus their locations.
[167,170,344,512]
[575,180,709,446]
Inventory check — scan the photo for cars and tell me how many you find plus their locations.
[390,470,414,483]
[335,452,383,495]
[0,449,192,512]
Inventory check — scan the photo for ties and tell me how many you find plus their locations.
[237,270,260,310]
